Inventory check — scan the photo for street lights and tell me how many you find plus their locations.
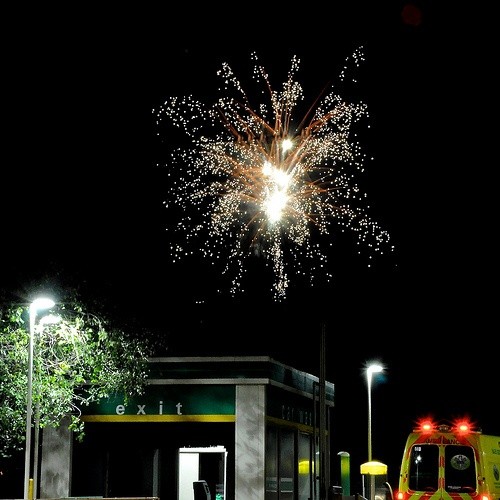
[24,296,55,498]
[366,365,383,500]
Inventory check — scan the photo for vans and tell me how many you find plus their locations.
[399,426,499,500]
